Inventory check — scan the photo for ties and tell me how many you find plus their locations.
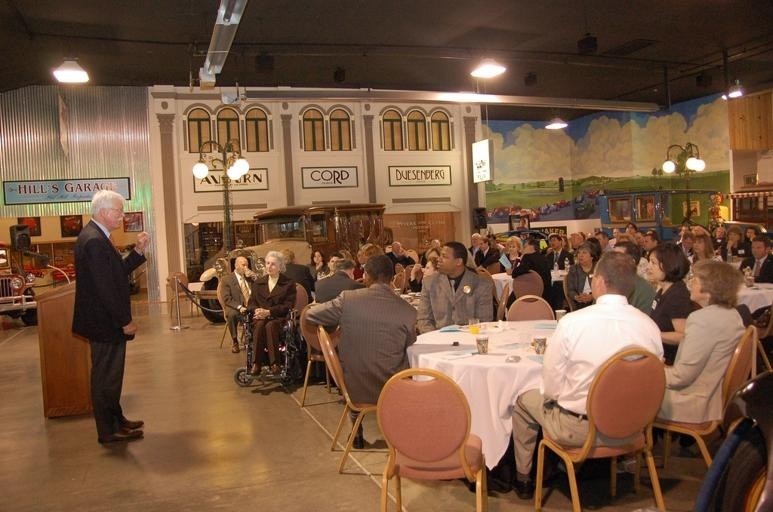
[754,258,763,278]
[451,278,456,295]
[555,251,559,262]
[108,233,120,252]
[239,279,251,307]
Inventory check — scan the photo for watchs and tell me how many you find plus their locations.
[236,304,243,310]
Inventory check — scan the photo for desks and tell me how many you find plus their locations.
[407,319,560,471]
[736,283,773,314]
[491,269,568,305]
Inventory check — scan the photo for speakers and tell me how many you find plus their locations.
[10,224,30,252]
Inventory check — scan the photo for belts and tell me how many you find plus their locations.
[551,401,589,421]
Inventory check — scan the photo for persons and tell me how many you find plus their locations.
[738,238,773,284]
[304,255,417,450]
[315,259,367,303]
[339,249,365,281]
[421,233,505,266]
[72,190,151,444]
[246,251,296,376]
[280,248,315,297]
[570,222,717,259]
[364,245,394,285]
[409,261,441,290]
[645,242,692,367]
[614,240,656,316]
[386,241,417,267]
[220,256,261,353]
[306,250,328,279]
[614,259,747,477]
[739,227,758,249]
[511,251,664,499]
[357,248,367,278]
[721,226,741,261]
[385,245,393,253]
[542,233,575,319]
[416,241,494,334]
[711,225,726,259]
[319,251,344,280]
[361,242,373,250]
[565,240,601,312]
[505,238,554,310]
[503,236,523,271]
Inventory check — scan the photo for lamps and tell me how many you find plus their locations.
[53,57,89,83]
[545,115,568,129]
[470,54,506,79]
[193,139,249,254]
[662,142,705,225]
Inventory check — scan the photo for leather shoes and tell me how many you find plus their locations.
[347,433,365,448]
[514,479,534,500]
[269,363,281,375]
[99,429,145,445]
[251,363,262,375]
[231,342,240,353]
[122,418,144,428]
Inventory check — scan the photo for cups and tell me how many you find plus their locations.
[746,276,754,287]
[476,337,488,355]
[555,310,566,323]
[534,338,546,354]
[468,319,479,336]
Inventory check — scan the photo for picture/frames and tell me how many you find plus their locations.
[17,217,42,237]
[60,215,83,237]
[3,177,131,206]
[123,211,143,233]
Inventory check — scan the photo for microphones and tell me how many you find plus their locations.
[23,250,39,257]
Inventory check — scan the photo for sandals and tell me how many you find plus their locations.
[615,459,636,474]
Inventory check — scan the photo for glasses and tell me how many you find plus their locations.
[110,208,125,214]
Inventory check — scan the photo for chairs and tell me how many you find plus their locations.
[404,265,414,288]
[535,348,666,512]
[513,270,544,302]
[393,272,405,295]
[497,282,509,322]
[217,282,244,349]
[395,263,404,274]
[316,325,396,480]
[294,283,308,314]
[300,303,346,407]
[754,301,773,372]
[377,368,487,512]
[635,326,757,491]
[506,295,554,321]
